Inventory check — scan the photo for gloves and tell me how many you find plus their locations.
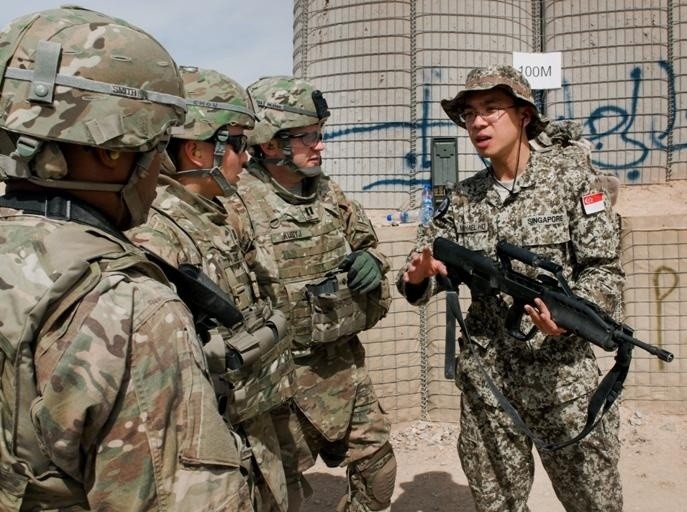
[340,250,383,297]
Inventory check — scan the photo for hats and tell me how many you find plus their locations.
[441,63,550,140]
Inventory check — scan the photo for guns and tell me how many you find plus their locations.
[433,236,674,363]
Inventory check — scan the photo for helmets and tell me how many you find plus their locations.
[246,75,331,155]
[167,64,260,141]
[0,4,189,195]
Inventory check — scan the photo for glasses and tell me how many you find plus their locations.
[292,131,323,146]
[212,131,248,155]
[457,101,519,123]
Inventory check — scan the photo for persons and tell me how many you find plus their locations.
[393,62,627,511]
[216,74,399,511]
[122,62,314,511]
[0,3,261,511]
